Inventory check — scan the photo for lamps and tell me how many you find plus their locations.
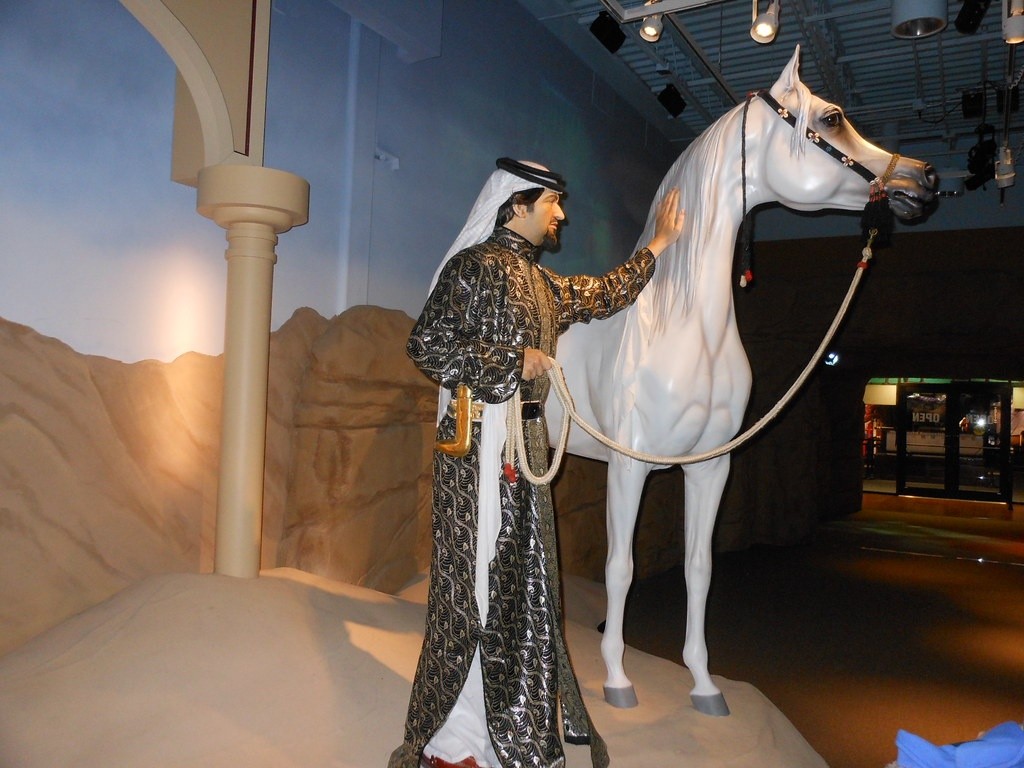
[1001,0,1024,44]
[750,0,782,44]
[995,146,1016,188]
[639,0,664,42]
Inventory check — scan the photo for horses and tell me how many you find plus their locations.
[542,42,939,717]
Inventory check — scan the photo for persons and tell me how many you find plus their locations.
[386,156,686,768]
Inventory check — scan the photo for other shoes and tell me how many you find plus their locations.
[420,753,481,768]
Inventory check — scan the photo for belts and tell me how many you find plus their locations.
[446,398,545,423]
